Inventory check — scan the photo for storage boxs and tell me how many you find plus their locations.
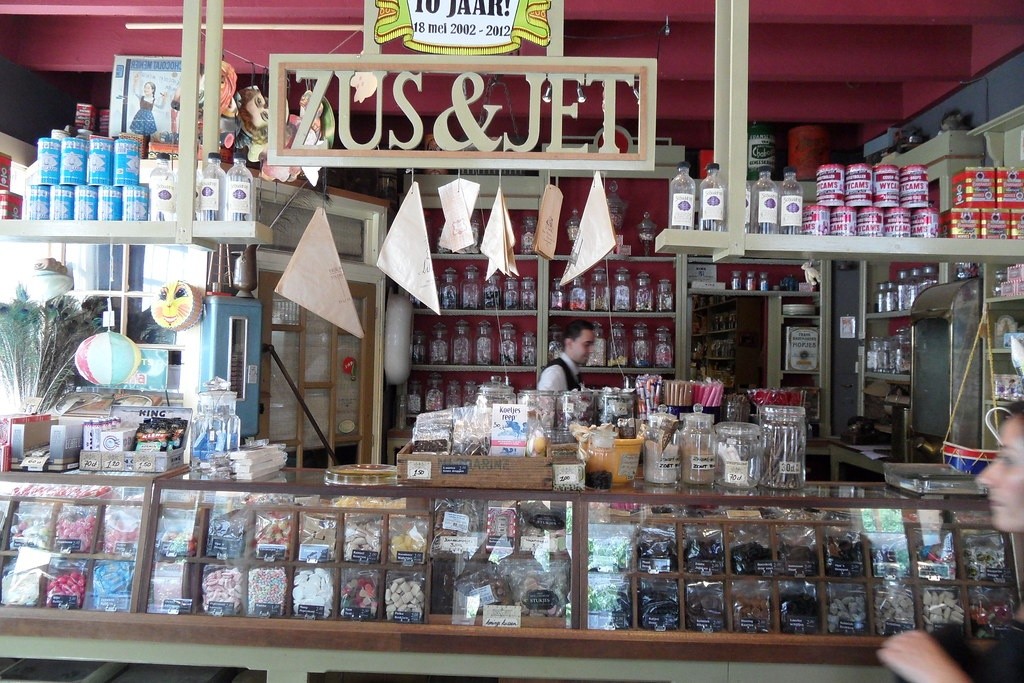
[688,264,726,290]
[75,103,109,136]
[1000,265,1024,297]
[784,387,820,419]
[10,405,193,473]
[397,439,554,490]
[784,325,820,372]
[939,167,1024,239]
[0,152,23,219]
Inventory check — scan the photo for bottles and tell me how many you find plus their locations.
[387,146,987,497]
[149,153,179,224]
[179,157,201,221]
[199,153,227,221]
[225,151,254,221]
[187,375,242,481]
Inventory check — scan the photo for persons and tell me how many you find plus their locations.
[877,399,1024,683]
[129,71,180,133]
[536,321,596,393]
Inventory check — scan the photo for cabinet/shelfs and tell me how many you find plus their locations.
[0,465,1023,683]
[856,130,986,416]
[396,254,683,430]
[767,290,826,436]
[692,296,763,393]
[966,105,1024,450]
